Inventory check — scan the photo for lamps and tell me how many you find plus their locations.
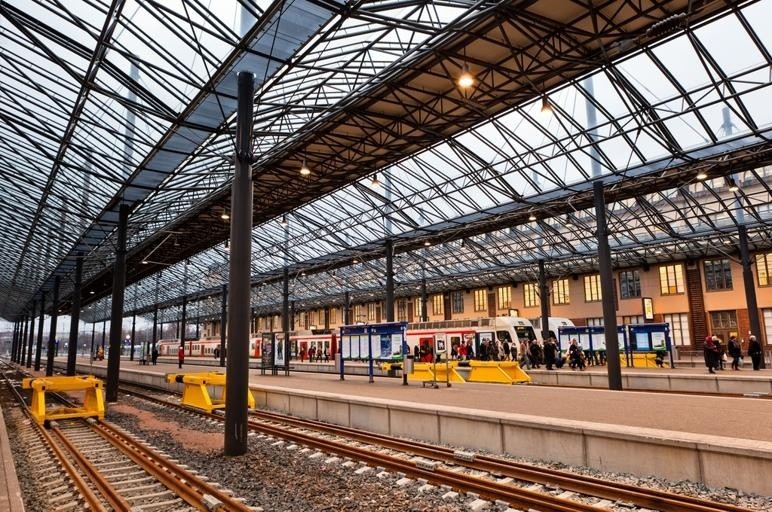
[224,240,231,252]
[458,41,475,90]
[299,140,311,177]
[538,77,556,115]
[695,163,707,180]
[221,200,231,220]
[728,177,738,194]
[367,153,379,191]
[282,214,288,227]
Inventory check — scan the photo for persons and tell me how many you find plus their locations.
[299,343,331,363]
[177,346,185,369]
[696,332,766,375]
[151,347,159,365]
[407,332,607,371]
[654,350,670,369]
[277,339,283,361]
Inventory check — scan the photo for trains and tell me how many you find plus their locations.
[156,317,578,365]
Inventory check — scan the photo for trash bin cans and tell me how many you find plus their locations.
[403,355,414,374]
[334,354,341,371]
